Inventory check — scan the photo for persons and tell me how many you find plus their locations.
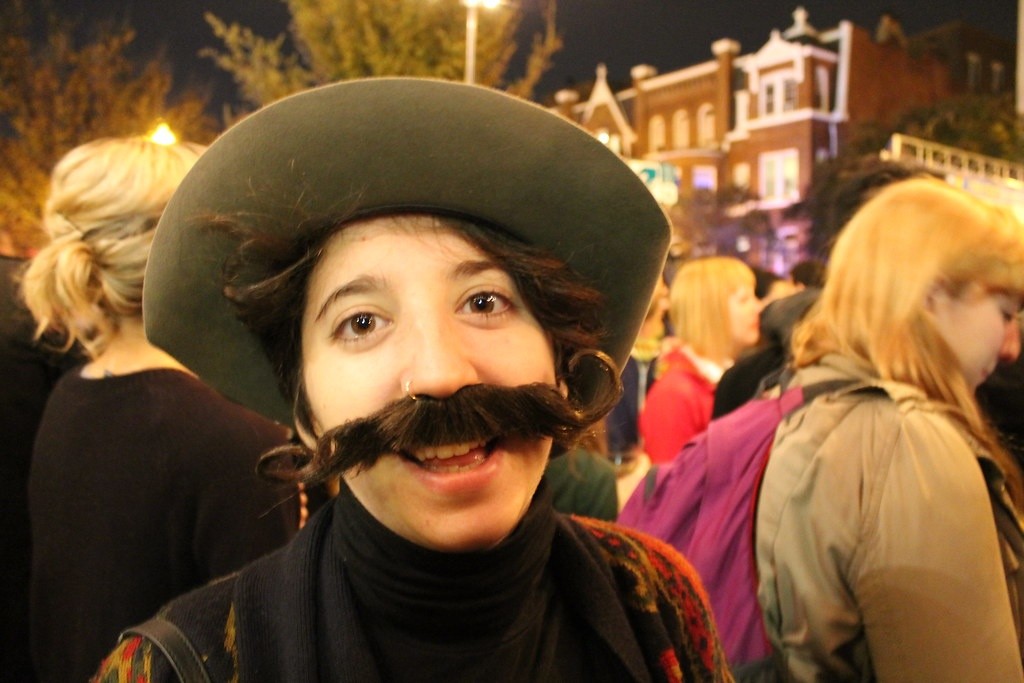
[1,75,1024,683]
[754,173,1024,683]
[89,75,737,683]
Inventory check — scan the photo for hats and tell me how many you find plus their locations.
[142,76,672,430]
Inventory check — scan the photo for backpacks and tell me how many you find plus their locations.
[616,379,856,683]
